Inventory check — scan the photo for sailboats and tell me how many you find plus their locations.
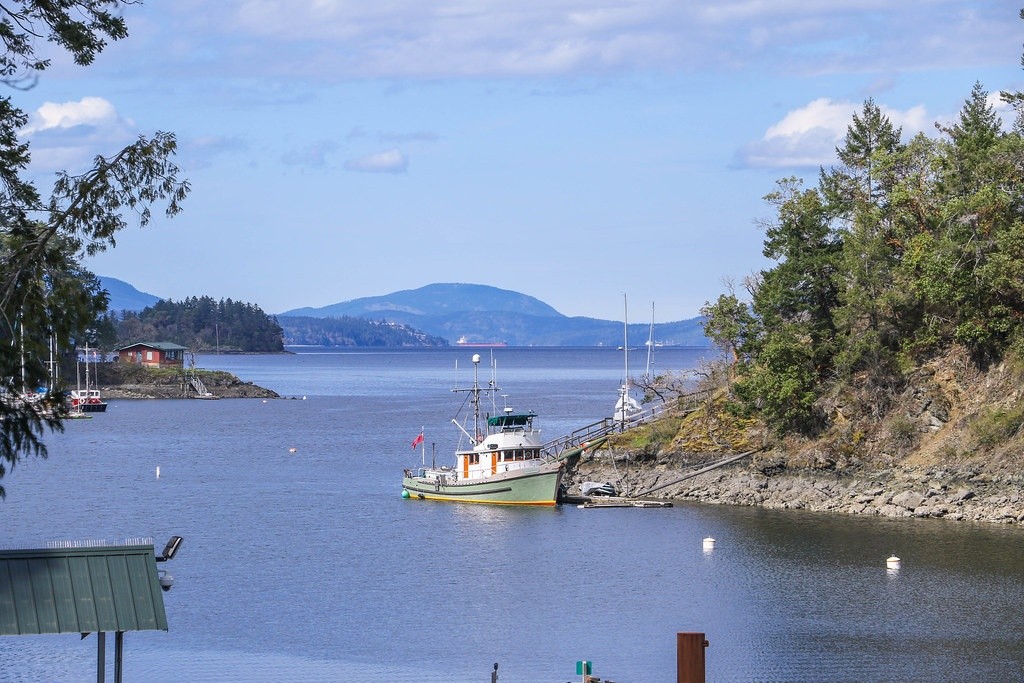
[640,301,666,394]
[612,292,644,422]
[0,322,107,412]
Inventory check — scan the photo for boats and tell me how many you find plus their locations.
[457,334,506,347]
[400,347,563,507]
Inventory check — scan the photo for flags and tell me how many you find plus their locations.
[412,433,423,449]
